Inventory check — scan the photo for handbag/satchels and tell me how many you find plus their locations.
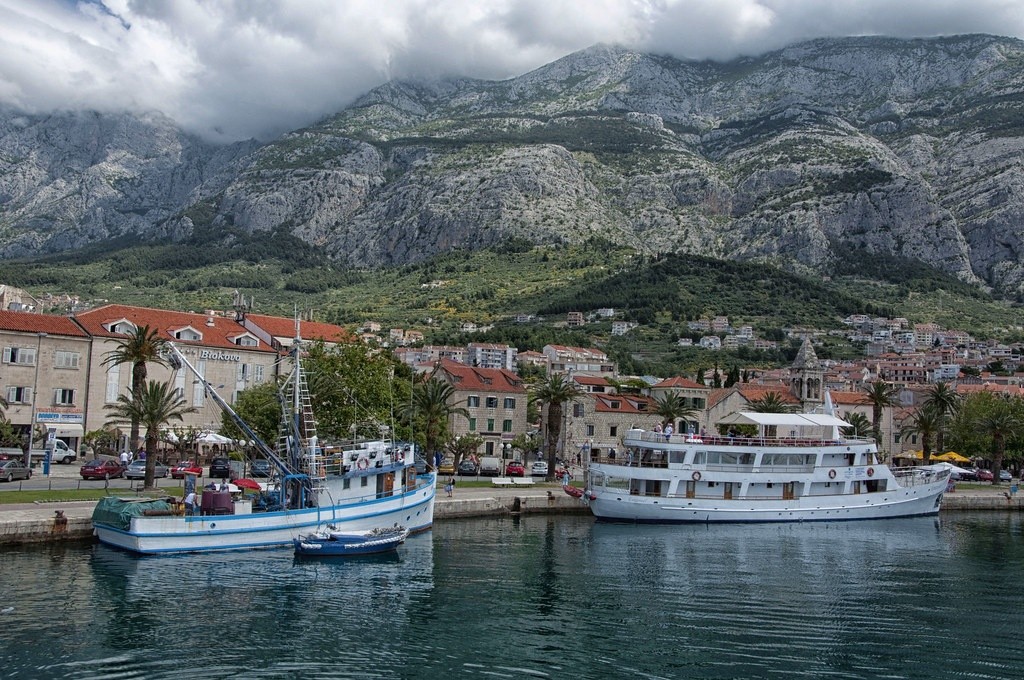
[444,485,450,492]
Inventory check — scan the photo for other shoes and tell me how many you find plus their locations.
[447,496,452,497]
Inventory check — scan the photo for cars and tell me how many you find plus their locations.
[504,461,524,477]
[210,457,231,478]
[458,460,479,476]
[79,458,124,481]
[0,459,33,482]
[437,459,456,476]
[531,461,549,477]
[250,458,271,478]
[123,459,170,480]
[170,461,203,479]
[950,466,1012,483]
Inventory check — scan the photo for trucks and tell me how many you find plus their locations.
[0,438,78,465]
[479,457,502,477]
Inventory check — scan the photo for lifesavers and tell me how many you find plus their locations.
[867,467,874,477]
[358,458,369,470]
[396,449,405,463]
[692,472,701,481]
[829,469,836,479]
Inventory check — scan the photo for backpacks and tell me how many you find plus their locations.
[452,479,455,485]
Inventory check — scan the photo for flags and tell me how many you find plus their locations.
[582,440,589,451]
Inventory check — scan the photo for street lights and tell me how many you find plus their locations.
[26,332,47,468]
[499,443,512,486]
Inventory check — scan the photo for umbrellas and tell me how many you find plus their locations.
[894,449,971,466]
[233,479,261,490]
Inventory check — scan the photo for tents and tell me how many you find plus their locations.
[164,430,233,465]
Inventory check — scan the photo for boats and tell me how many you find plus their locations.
[85,292,437,558]
[558,410,956,527]
[291,520,412,559]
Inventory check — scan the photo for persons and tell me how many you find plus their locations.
[624,447,639,467]
[119,450,128,467]
[562,465,571,486]
[184,492,199,516]
[700,425,707,441]
[286,494,291,503]
[220,479,229,492]
[573,452,581,466]
[1010,483,1018,496]
[656,422,662,442]
[537,450,543,461]
[688,425,695,444]
[608,448,615,464]
[447,478,455,497]
[212,444,226,455]
[665,423,673,443]
[139,447,146,459]
[210,482,216,490]
[555,451,560,463]
[128,449,133,465]
